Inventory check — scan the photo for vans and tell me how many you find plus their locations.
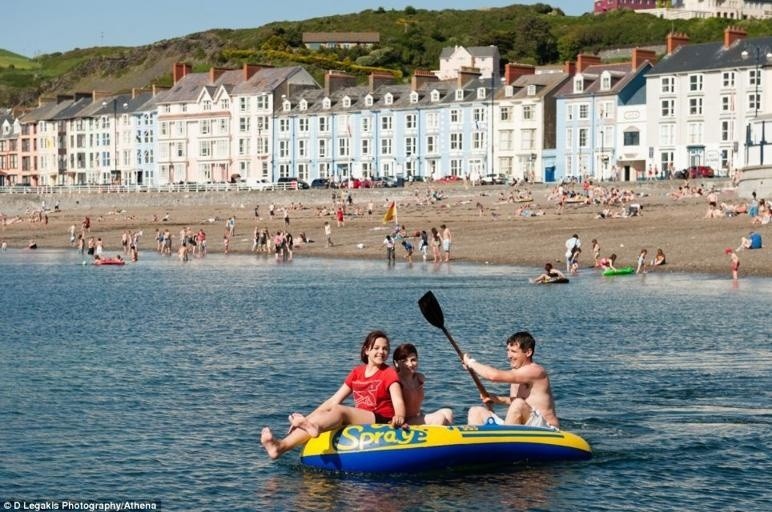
[276,177,309,189]
[246,178,275,191]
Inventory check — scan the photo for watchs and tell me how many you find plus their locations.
[467,359,475,368]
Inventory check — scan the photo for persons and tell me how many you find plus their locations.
[258,328,408,460]
[0,161,771,288]
[261,458,561,512]
[461,329,563,431]
[386,342,455,428]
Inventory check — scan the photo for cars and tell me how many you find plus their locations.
[339,172,433,197]
[11,183,32,194]
[436,176,463,183]
[310,179,336,189]
[480,175,505,184]
[678,165,713,178]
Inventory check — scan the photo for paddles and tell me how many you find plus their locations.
[416,289,495,415]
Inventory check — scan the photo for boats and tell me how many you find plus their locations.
[301,417,591,472]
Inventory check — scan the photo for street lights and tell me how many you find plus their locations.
[261,84,287,179]
[479,71,507,173]
[100,95,129,181]
[739,44,772,118]
